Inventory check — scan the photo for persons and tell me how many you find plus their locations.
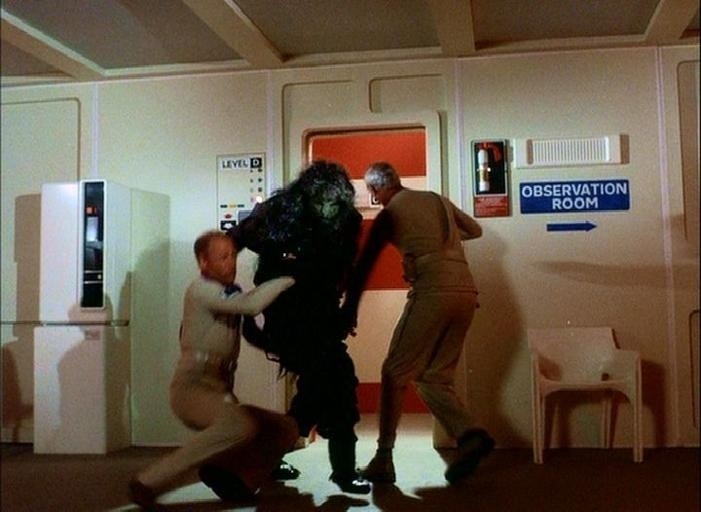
[331,159,499,486]
[124,230,303,511]
[223,159,373,494]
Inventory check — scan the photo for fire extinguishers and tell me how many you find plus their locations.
[476,142,502,192]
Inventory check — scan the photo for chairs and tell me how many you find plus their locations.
[527,328,644,465]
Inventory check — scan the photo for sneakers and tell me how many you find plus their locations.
[273,460,299,481]
[356,455,396,484]
[445,427,496,483]
[328,471,371,494]
[127,480,167,511]
[250,480,297,497]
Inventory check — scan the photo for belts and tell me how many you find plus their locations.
[192,351,238,373]
[415,250,467,269]
